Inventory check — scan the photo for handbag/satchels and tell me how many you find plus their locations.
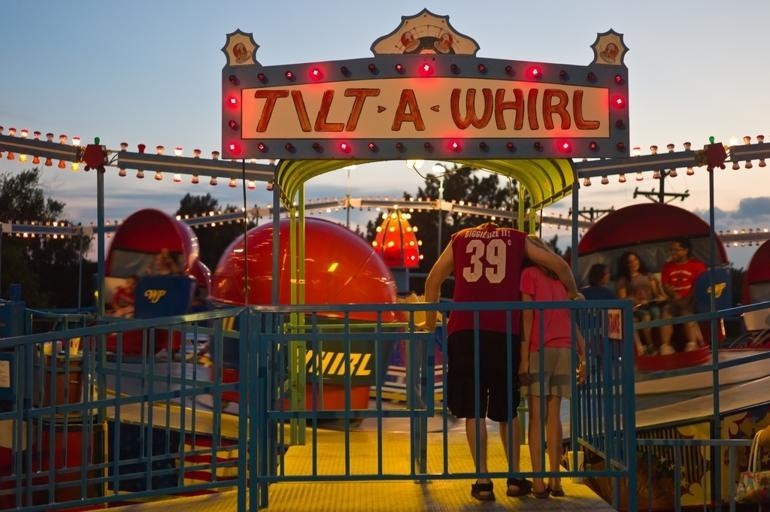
[734,430,770,504]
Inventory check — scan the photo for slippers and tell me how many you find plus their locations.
[534,482,564,498]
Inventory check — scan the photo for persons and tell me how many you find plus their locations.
[615,251,667,354]
[111,274,137,319]
[147,247,180,275]
[577,262,624,381]
[424,221,586,502]
[520,233,587,495]
[756,424,770,512]
[655,237,710,355]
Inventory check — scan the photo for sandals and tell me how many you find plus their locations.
[472,480,495,500]
[507,478,532,496]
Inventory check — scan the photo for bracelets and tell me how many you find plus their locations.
[574,293,581,300]
[579,359,585,364]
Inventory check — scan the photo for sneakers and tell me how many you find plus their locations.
[156,350,182,363]
[636,342,696,357]
